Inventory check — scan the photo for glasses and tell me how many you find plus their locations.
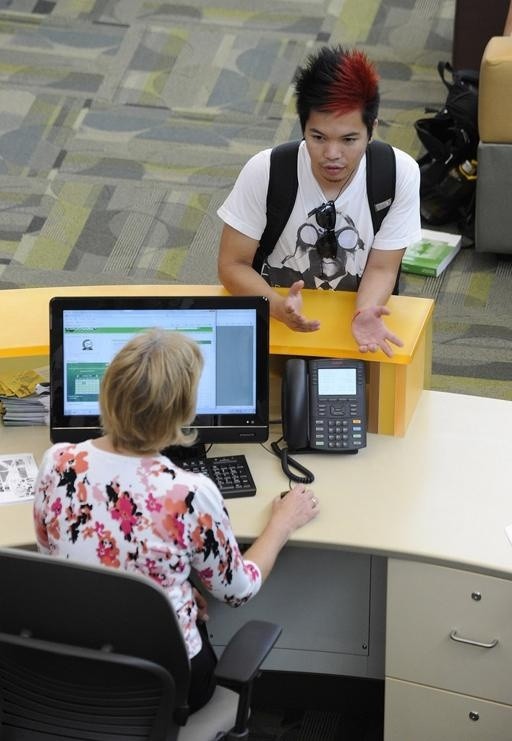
[316,201,337,260]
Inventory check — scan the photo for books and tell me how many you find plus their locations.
[0,365,49,426]
[401,226,462,278]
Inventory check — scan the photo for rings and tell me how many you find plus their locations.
[312,497,317,505]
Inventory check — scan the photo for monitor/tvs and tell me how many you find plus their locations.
[50,296,268,458]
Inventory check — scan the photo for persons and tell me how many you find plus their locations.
[32,325,322,728]
[214,41,424,357]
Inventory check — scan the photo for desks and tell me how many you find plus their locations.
[3,390,512,740]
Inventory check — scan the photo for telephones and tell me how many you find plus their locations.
[281,358,366,454]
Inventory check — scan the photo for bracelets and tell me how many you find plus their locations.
[352,311,361,321]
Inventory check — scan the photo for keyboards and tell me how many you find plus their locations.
[172,454,259,498]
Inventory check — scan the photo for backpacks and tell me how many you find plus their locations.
[413,59,480,227]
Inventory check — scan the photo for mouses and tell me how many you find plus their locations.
[282,489,304,498]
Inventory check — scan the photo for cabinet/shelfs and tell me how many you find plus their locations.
[382,557,512,738]
